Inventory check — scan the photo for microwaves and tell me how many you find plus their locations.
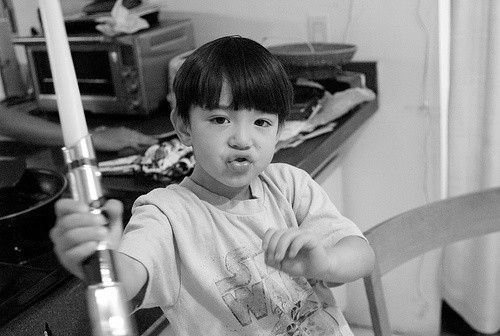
[10,17,197,117]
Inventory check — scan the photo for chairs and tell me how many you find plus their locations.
[363,188,500,336]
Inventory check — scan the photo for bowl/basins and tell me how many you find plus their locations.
[0,166,67,234]
[266,42,356,67]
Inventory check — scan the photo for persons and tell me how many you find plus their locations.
[49,36,376,335]
